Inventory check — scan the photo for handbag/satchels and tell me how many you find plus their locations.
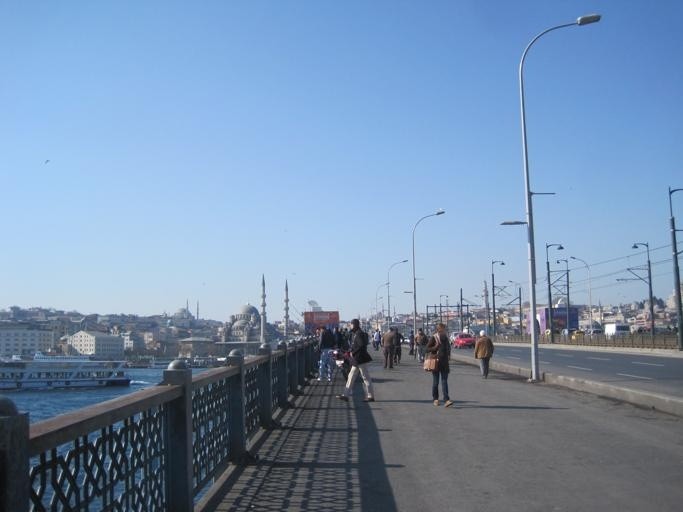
[351,352,369,366]
[424,354,440,373]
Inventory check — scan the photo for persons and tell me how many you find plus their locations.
[313,324,354,384]
[425,324,452,408]
[363,326,430,369]
[475,330,494,379]
[336,319,375,402]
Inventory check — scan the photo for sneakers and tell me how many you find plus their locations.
[336,395,349,401]
[364,398,375,402]
[434,400,439,406]
[445,401,453,407]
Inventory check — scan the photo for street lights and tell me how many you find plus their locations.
[412,207,447,336]
[495,9,602,383]
[372,238,658,337]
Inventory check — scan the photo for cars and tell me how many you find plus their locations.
[399,337,409,343]
[449,331,460,344]
[451,333,475,350]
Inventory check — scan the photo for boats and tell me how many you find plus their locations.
[0,348,131,391]
[121,354,218,369]
